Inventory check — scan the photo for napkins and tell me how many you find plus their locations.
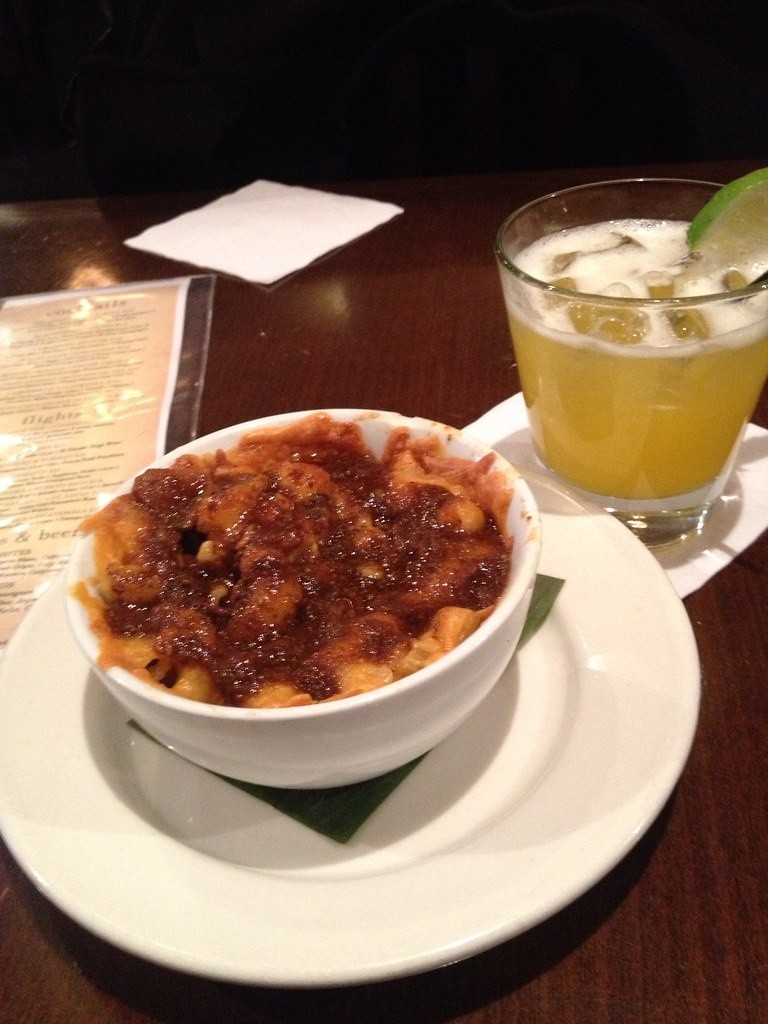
[123,180,405,285]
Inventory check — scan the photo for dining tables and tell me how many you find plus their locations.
[0,156,768,1024]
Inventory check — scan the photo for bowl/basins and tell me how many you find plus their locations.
[64,409,539,789]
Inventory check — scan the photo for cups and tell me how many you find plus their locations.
[494,178,768,546]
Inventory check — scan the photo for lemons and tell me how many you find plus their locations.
[684,167,768,268]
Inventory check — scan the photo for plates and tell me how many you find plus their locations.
[0,470,701,990]
